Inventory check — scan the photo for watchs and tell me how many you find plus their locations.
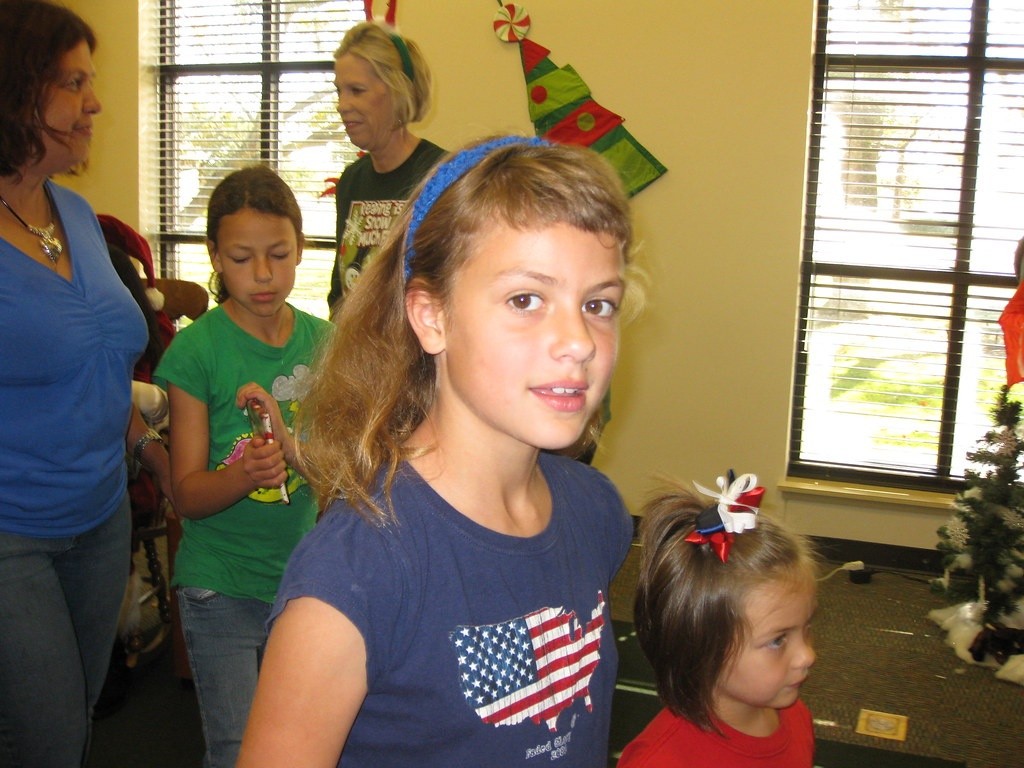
[134,428,164,464]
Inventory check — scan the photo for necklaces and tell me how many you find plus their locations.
[0,195,63,262]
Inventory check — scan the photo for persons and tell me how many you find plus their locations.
[0,0,173,768]
[326,23,448,309]
[233,135,634,768]
[615,470,818,768]
[152,165,335,768]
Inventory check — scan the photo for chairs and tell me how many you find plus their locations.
[129,277,207,692]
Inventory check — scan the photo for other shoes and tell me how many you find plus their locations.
[90,640,135,722]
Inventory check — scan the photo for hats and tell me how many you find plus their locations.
[98,215,165,311]
